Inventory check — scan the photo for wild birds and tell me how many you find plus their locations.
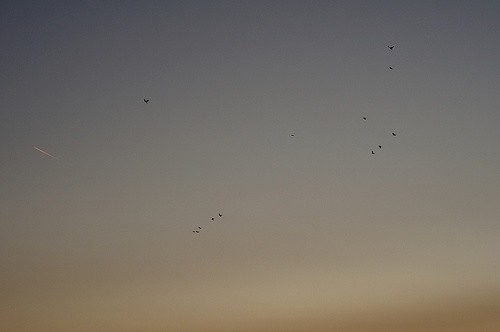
[192,213,223,234]
[389,66,392,70]
[363,117,366,120]
[379,145,381,148]
[392,132,396,136]
[371,150,375,154]
[144,98,149,103]
[387,46,394,50]
